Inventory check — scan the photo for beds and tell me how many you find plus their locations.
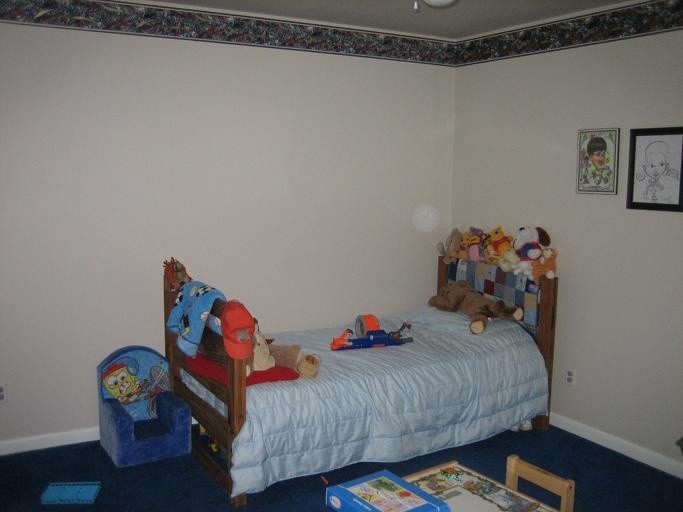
[162,224,558,511]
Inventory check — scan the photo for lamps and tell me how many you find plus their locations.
[423,0,456,8]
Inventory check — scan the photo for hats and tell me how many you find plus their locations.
[222,300,255,360]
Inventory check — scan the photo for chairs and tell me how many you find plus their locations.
[95,345,192,468]
[507,447,576,511]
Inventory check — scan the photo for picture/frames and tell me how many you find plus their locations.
[575,127,618,195]
[625,126,682,211]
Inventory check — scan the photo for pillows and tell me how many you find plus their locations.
[186,336,301,387]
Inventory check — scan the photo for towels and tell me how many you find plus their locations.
[165,280,227,359]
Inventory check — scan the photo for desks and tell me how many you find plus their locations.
[401,459,564,512]
